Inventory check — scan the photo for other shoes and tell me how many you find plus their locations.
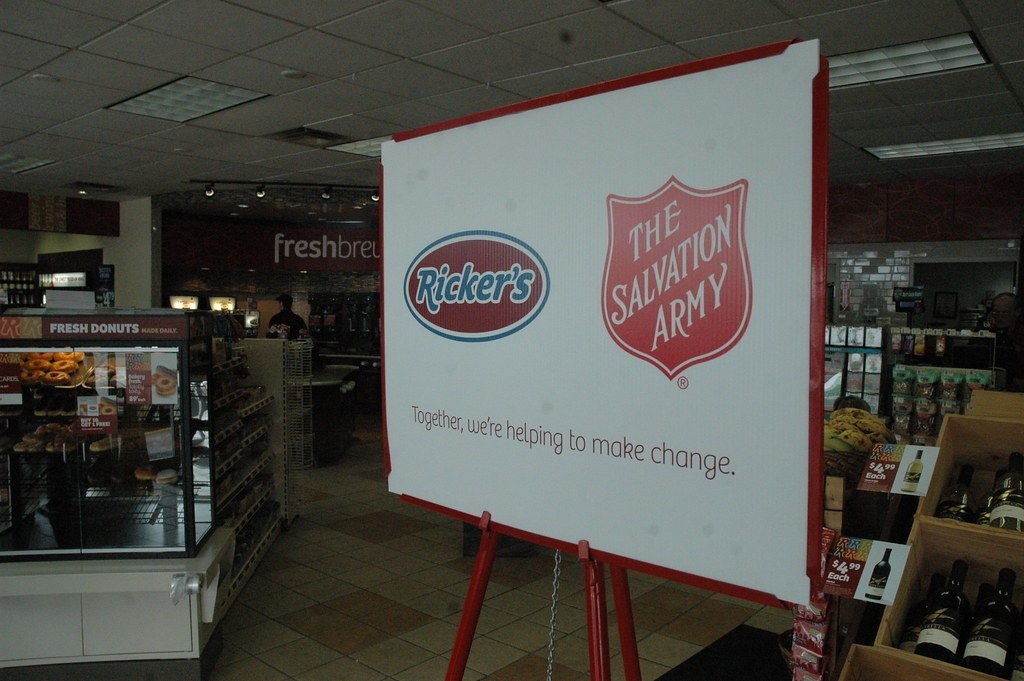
[464,539,534,556]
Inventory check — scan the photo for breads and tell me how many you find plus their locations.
[14,424,125,452]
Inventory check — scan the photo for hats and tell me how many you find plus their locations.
[275,294,293,304]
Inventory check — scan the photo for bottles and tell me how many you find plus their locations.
[97,296,103,307]
[0,271,34,289]
[10,294,33,304]
[896,559,1024,681]
[901,450,924,492]
[865,548,892,600]
[937,453,1024,532]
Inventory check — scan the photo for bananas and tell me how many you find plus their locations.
[823,408,896,454]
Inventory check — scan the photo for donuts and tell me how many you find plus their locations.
[134,464,179,485]
[155,376,177,395]
[15,351,118,387]
[102,405,114,415]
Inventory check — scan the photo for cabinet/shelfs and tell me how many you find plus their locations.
[820,304,1023,681]
[0,261,380,669]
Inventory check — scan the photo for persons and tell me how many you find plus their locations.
[266,293,310,340]
[990,292,1024,345]
[245,301,260,338]
